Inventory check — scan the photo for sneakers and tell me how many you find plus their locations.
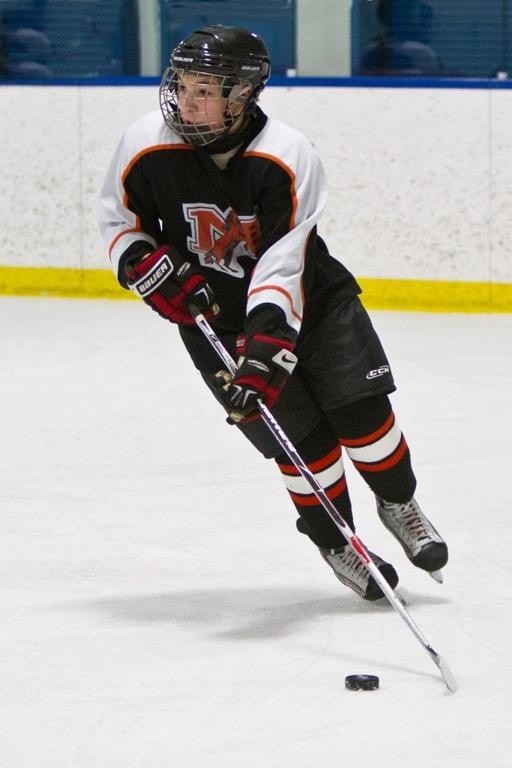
[371,489,451,575]
[317,534,401,601]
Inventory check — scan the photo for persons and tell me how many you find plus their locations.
[6,25,56,80]
[350,1,452,78]
[94,20,449,599]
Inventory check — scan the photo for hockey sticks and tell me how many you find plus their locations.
[185,299,461,694]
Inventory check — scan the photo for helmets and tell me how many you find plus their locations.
[160,23,271,150]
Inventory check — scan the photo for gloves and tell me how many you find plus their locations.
[124,243,223,327]
[213,328,300,427]
